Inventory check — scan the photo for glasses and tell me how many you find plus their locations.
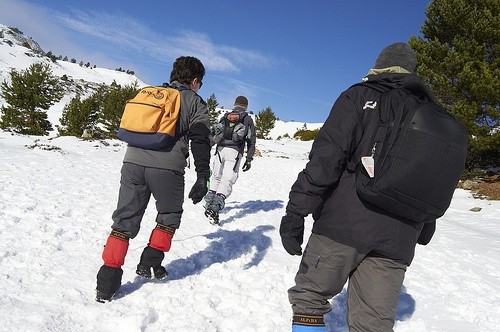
[197,78,202,89]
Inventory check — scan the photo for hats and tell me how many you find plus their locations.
[372,42,417,73]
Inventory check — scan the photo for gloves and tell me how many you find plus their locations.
[189,177,210,204]
[418,220,437,245]
[242,160,251,172]
[280,229,304,256]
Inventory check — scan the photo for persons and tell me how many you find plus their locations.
[95,56,212,304]
[204,96,257,226]
[279,42,442,332]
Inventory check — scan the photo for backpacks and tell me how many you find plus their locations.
[116,83,190,151]
[355,80,469,224]
[219,110,249,146]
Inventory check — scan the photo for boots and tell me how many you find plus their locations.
[203,190,226,227]
[136,246,169,280]
[95,265,123,303]
